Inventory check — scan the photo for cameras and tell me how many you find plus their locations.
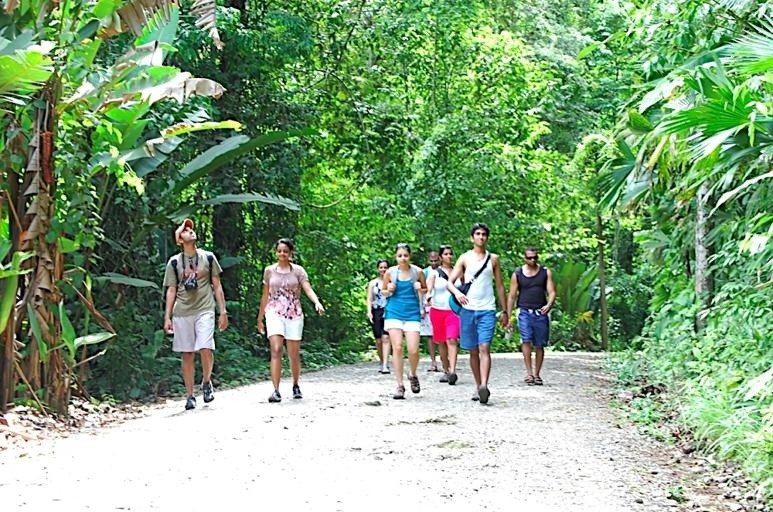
[185,279,198,290]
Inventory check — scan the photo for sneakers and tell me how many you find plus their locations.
[293,384,303,398]
[394,385,405,399]
[408,371,421,393]
[185,394,196,410]
[269,391,282,402]
[201,380,215,402]
[472,385,489,403]
[378,364,390,373]
[428,366,457,384]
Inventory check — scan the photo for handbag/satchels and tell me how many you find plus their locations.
[449,283,471,313]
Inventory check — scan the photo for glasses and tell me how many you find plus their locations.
[525,256,537,259]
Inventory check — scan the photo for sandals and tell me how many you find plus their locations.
[525,375,542,386]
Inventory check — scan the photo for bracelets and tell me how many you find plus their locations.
[501,310,507,314]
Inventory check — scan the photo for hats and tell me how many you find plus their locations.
[175,218,193,244]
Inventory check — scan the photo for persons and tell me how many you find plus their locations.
[419,251,449,371]
[506,248,556,386]
[256,238,324,402]
[163,219,228,410]
[367,260,391,374]
[425,245,464,385]
[446,224,508,404]
[382,244,428,400]
